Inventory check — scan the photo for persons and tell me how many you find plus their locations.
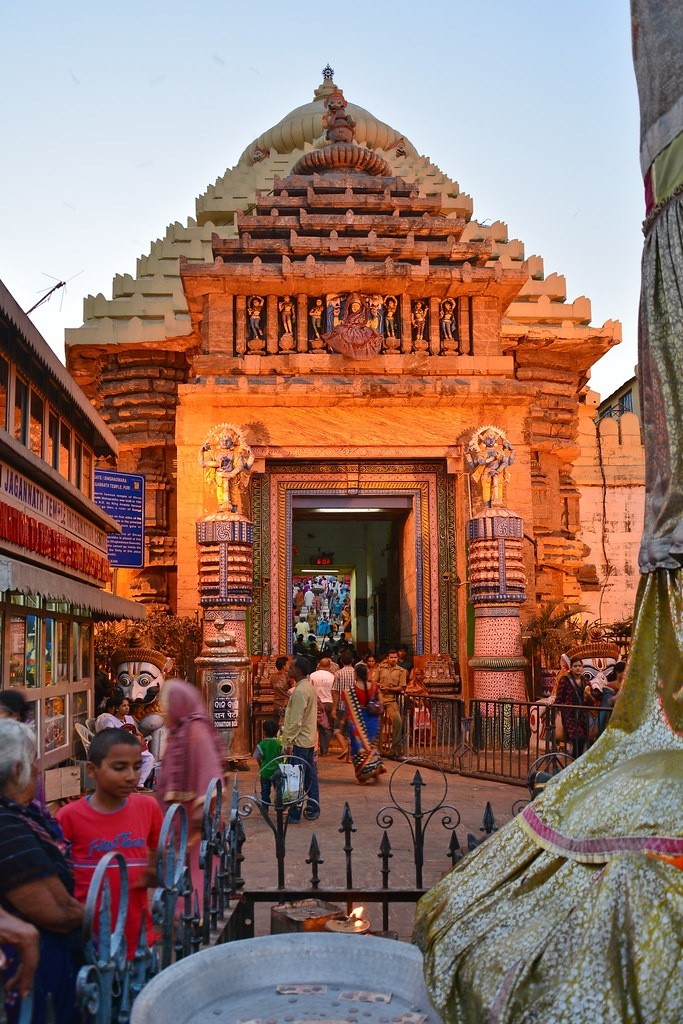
[440,298,457,340]
[553,657,628,761]
[556,626,621,740]
[0,689,46,806]
[271,575,429,761]
[340,664,387,785]
[281,657,320,823]
[156,679,227,942]
[253,90,408,163]
[247,295,265,340]
[411,299,429,340]
[109,632,173,732]
[253,720,283,822]
[94,696,155,793]
[56,727,174,963]
[0,718,86,1023]
[0,909,40,999]
[309,294,398,360]
[198,423,254,514]
[467,428,514,508]
[278,296,296,336]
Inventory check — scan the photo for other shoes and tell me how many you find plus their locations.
[283,817,298,824]
[354,779,367,785]
[321,753,330,757]
[337,749,348,760]
[372,768,388,778]
[137,786,154,793]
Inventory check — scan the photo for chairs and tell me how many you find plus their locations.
[75,717,96,757]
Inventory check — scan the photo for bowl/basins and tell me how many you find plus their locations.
[128,932,445,1024]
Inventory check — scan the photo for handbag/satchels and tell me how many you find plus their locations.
[368,682,381,714]
[278,753,303,804]
